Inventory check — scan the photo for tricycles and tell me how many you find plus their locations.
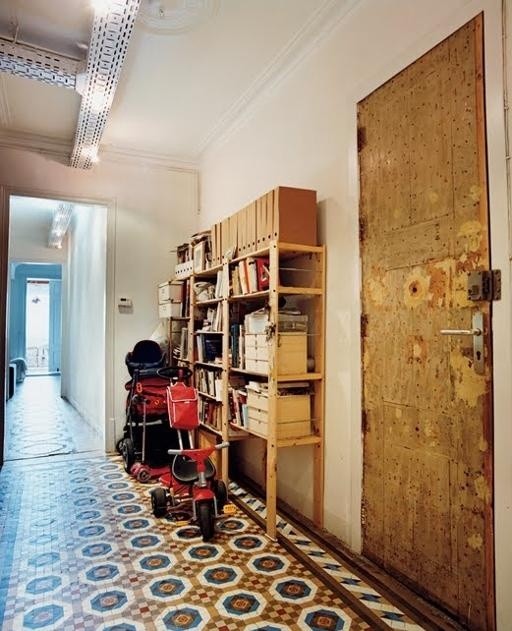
[150,366,232,543]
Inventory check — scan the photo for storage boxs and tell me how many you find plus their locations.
[159,303,181,319]
[244,334,307,376]
[158,281,184,305]
[246,391,311,439]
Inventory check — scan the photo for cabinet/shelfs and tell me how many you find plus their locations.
[167,241,326,542]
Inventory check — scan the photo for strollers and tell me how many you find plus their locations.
[115,341,192,485]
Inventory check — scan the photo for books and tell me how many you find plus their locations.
[190,254,270,442]
